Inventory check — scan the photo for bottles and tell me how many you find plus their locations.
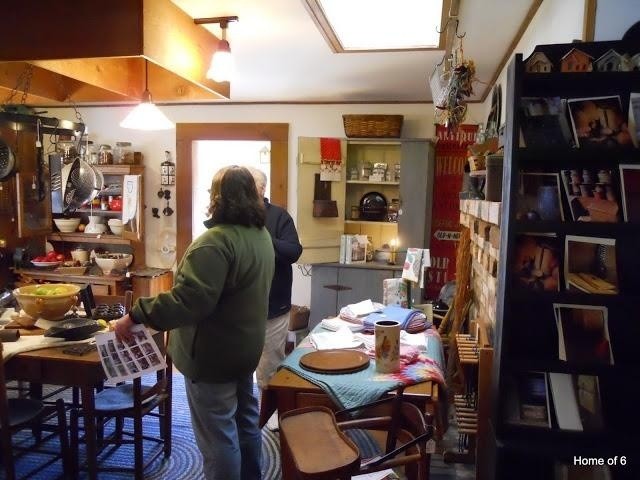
[593,243,610,281]
[366,235,374,262]
[348,160,400,182]
[87,196,122,210]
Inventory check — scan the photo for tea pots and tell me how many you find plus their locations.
[70,248,90,262]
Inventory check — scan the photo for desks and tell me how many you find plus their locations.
[258,300,447,479]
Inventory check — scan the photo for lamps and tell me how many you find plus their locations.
[194,15,240,83]
[119,60,176,131]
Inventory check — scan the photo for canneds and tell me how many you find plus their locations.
[97,145,113,165]
[350,206,360,220]
[113,142,134,165]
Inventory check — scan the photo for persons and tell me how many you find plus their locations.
[507,235,559,292]
[246,168,302,432]
[114,165,276,480]
[572,100,632,147]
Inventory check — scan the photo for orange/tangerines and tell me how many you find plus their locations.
[32,287,67,296]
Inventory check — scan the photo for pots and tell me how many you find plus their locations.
[62,155,104,214]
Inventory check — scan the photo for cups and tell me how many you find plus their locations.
[566,171,615,202]
[485,153,502,201]
[351,205,360,221]
[388,197,400,222]
[372,320,400,373]
[469,175,484,200]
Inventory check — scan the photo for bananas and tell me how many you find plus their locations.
[97,319,107,329]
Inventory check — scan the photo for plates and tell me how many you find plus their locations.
[361,191,386,221]
[300,349,369,374]
[30,260,61,266]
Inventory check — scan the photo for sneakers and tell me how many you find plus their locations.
[267,413,279,431]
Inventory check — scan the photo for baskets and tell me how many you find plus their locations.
[468,155,486,171]
[342,114,404,138]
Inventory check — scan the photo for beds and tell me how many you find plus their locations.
[0,308,166,480]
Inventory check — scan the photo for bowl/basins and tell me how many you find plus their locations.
[10,282,80,322]
[96,253,134,275]
[52,218,81,232]
[88,215,104,224]
[107,218,123,235]
[375,249,390,262]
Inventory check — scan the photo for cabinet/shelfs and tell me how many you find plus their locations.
[443,314,494,480]
[341,138,402,263]
[13,163,145,297]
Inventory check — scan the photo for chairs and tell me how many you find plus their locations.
[69,352,172,480]
[0,329,69,480]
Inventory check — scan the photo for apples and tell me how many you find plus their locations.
[77,224,86,232]
[33,251,64,263]
